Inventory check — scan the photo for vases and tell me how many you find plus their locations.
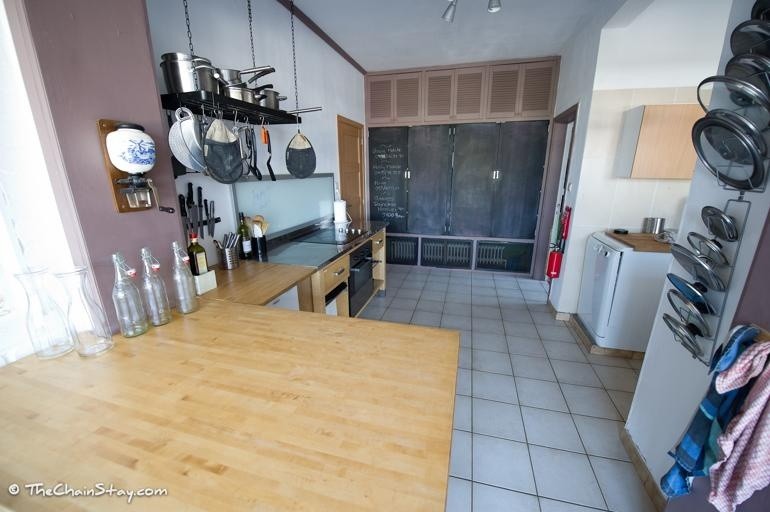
[105,123,157,174]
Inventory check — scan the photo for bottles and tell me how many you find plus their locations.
[109,251,147,339]
[251,235,268,258]
[11,265,72,362]
[236,210,254,261]
[136,245,173,327]
[187,232,209,276]
[56,265,116,360]
[170,239,199,319]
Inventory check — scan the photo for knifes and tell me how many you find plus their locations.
[195,185,206,242]
[178,193,192,247]
[204,198,215,238]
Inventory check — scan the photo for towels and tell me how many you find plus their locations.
[657,322,770,511]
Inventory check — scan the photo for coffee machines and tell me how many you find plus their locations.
[97,118,177,217]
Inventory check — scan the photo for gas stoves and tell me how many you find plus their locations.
[290,227,369,246]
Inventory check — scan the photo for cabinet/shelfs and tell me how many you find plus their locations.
[613,103,709,180]
[370,228,386,294]
[364,55,562,280]
[312,254,352,317]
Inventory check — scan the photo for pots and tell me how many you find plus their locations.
[157,52,288,111]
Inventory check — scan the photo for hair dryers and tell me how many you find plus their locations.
[334,199,347,222]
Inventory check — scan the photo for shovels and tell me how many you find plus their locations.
[266,131,276,181]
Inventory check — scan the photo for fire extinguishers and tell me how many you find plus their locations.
[547,242,562,278]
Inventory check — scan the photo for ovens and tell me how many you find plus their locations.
[347,239,375,319]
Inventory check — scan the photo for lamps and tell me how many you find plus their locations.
[488,0,502,14]
[441,0,458,24]
